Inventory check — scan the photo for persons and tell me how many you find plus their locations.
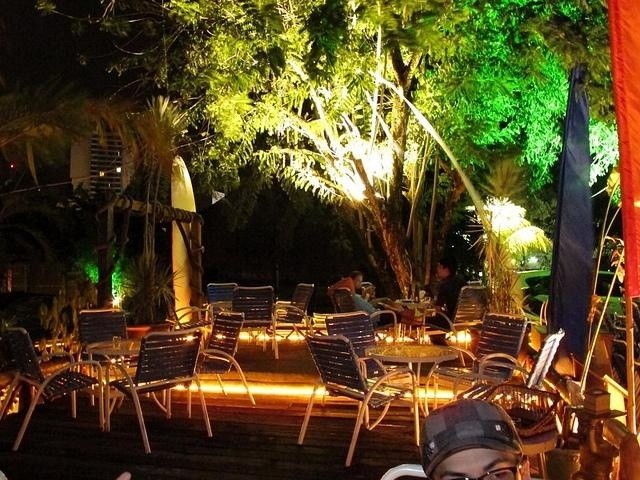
[422,258,466,329]
[337,276,392,326]
[350,270,376,300]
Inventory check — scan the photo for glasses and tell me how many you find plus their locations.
[451,457,519,480]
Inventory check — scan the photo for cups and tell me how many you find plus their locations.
[112,336,121,352]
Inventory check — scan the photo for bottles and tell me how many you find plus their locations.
[407,283,413,299]
[415,284,421,302]
